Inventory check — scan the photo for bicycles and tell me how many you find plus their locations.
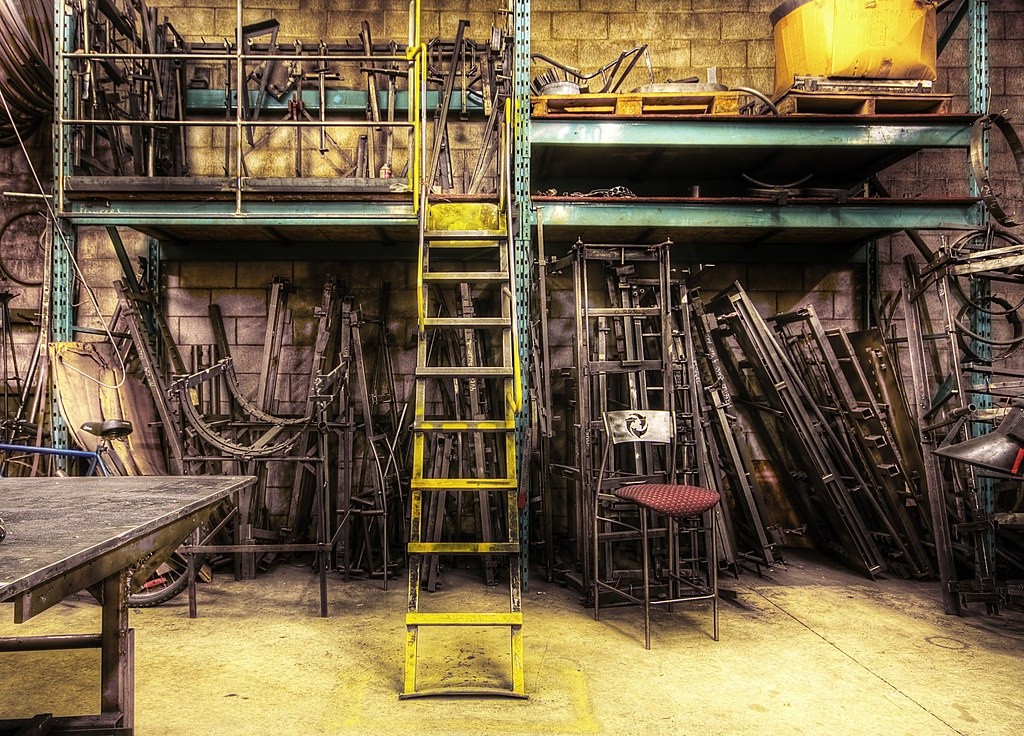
[1,419,210,608]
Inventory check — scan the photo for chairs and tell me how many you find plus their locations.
[591,407,723,651]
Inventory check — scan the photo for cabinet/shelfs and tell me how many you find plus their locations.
[37,0,1002,613]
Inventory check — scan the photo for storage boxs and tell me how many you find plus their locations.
[767,1,940,105]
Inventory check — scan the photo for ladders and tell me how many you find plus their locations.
[395,194,535,703]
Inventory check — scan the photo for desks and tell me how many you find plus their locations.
[0,469,263,736]
